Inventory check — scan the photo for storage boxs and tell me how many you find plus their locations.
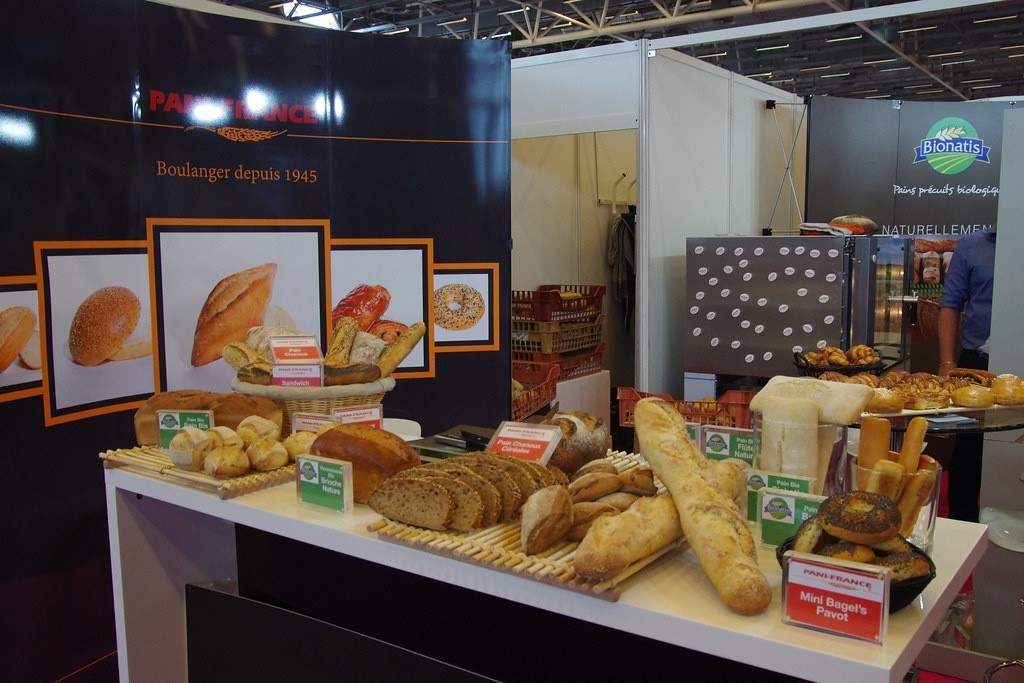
[511,284,759,428]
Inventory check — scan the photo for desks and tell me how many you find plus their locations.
[846,405,1024,434]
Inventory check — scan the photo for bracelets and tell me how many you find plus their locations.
[938,361,956,366]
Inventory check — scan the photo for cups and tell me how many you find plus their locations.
[753,414,849,499]
[850,456,942,556]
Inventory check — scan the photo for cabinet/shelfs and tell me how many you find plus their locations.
[104,423,990,683]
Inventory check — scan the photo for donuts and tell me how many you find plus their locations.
[786,489,931,583]
[434,284,485,330]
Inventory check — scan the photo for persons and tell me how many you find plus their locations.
[938,223,997,523]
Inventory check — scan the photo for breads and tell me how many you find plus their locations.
[512,291,586,317]
[309,422,421,505]
[801,345,1024,413]
[134,388,283,446]
[332,283,409,343]
[0,305,41,374]
[748,376,874,495]
[830,214,880,235]
[222,316,426,387]
[169,414,342,479]
[70,286,153,366]
[912,239,960,285]
[191,262,278,367]
[857,417,938,537]
[367,378,773,616]
